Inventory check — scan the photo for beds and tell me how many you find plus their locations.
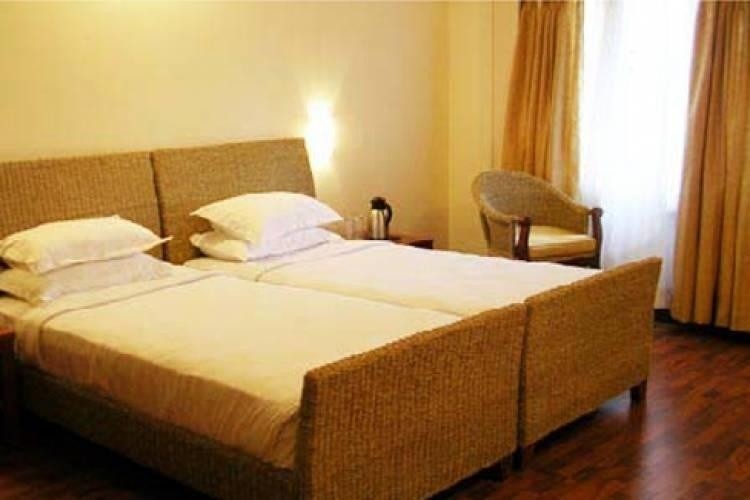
[1,150,531,500]
[152,138,661,458]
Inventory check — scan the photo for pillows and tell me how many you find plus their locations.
[184,190,347,258]
[1,251,179,311]
[189,225,343,265]
[1,214,177,273]
